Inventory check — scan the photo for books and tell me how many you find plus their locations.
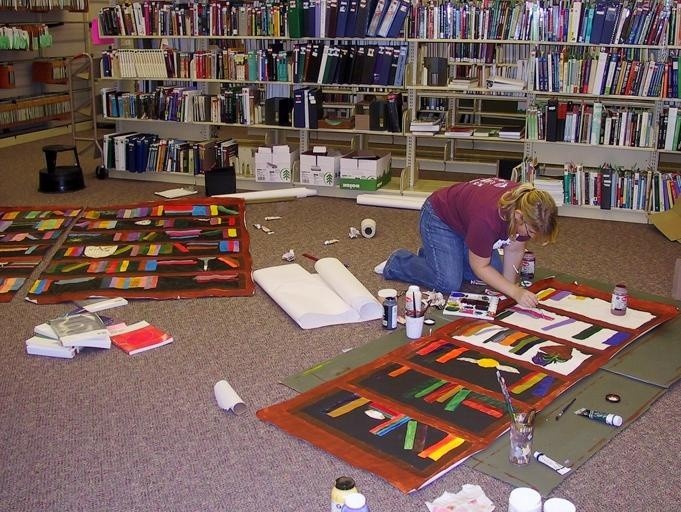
[97,0,290,175]
[408,0,533,139]
[288,1,409,133]
[537,0,680,212]
[26,299,173,359]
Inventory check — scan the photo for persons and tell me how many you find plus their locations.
[375,176,559,309]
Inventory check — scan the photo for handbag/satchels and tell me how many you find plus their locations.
[205,166,237,197]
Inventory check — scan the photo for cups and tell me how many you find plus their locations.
[508,486,544,512]
[405,311,425,340]
[510,416,534,467]
[543,498,577,512]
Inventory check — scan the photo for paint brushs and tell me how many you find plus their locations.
[513,265,544,319]
[496,369,519,423]
[555,397,577,424]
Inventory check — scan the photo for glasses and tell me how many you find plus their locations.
[524,220,533,240]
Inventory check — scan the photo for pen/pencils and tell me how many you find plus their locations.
[412,291,418,318]
[422,300,432,316]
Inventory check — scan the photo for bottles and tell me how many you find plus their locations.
[406,285,422,314]
[331,476,358,512]
[383,297,397,330]
[341,493,369,512]
[611,283,628,317]
[519,252,535,280]
